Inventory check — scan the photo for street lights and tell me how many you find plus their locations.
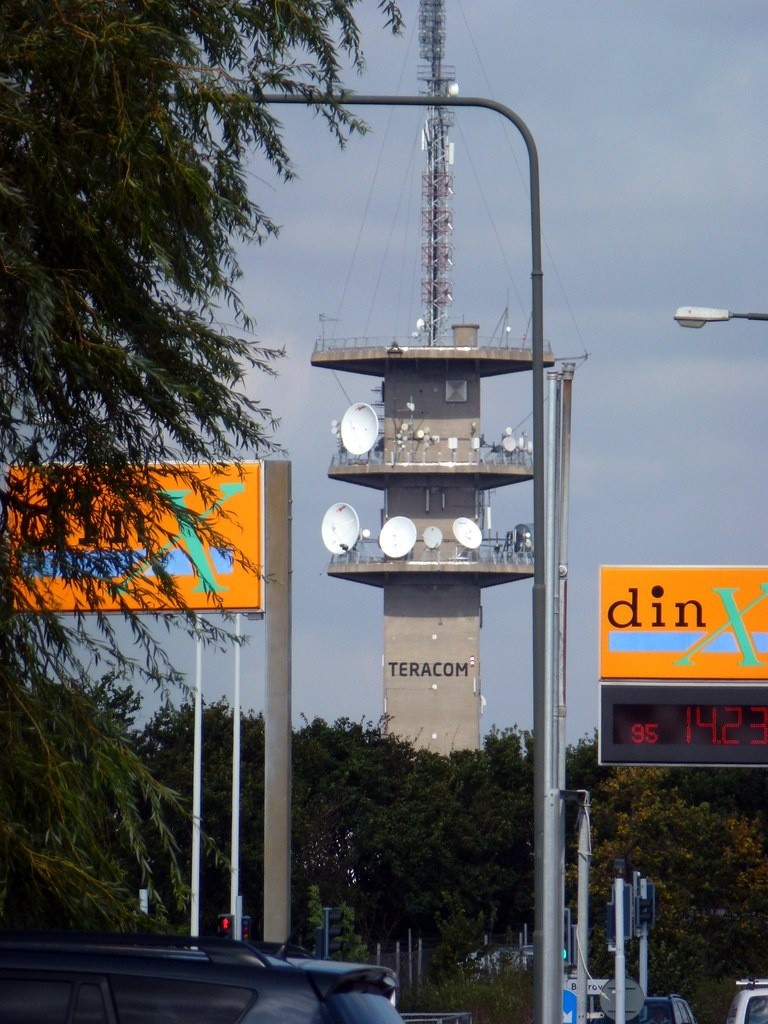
[323,907,331,959]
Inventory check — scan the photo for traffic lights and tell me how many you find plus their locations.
[219,913,234,940]
[241,916,251,943]
[562,910,571,968]
[636,896,651,926]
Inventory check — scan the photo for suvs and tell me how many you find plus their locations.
[1,939,403,1024]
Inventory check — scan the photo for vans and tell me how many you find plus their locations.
[624,995,696,1024]
[724,978,768,1024]
